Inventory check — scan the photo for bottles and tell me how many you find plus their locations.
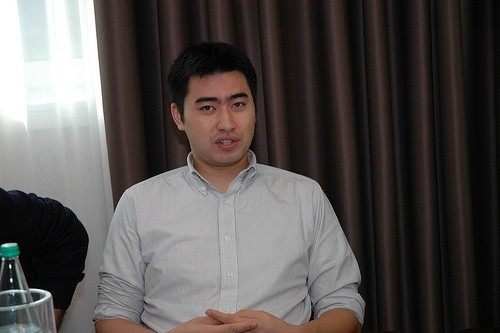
[0,243,44,333]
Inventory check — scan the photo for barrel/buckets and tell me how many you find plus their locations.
[0,289,56,333]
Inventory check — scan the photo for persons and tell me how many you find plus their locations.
[92,42,366,333]
[0,187,89,333]
